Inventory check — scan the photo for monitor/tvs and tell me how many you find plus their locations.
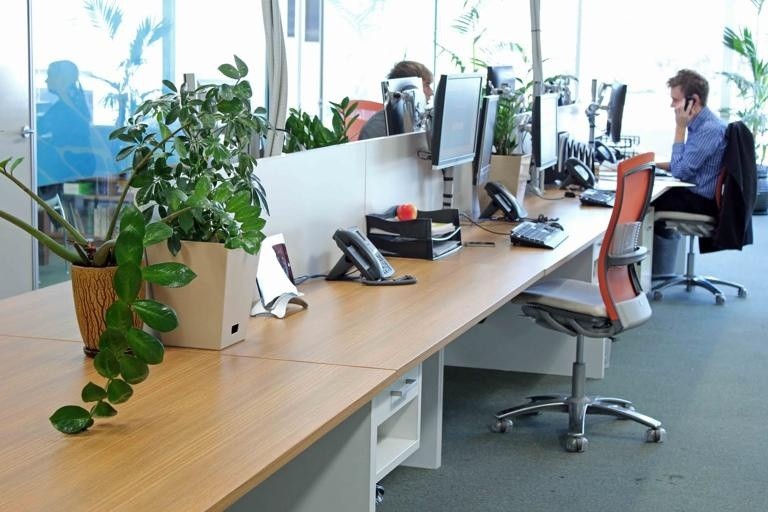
[605,84,627,143]
[380,76,427,136]
[533,94,558,172]
[431,74,485,173]
[487,66,515,97]
[473,95,499,186]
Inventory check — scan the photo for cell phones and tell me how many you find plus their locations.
[684,96,696,115]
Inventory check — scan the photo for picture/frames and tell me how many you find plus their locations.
[251,234,308,319]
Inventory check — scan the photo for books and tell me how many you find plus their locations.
[99,174,116,195]
[52,197,121,240]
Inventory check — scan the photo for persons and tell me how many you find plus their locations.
[653,68,726,215]
[36,60,97,228]
[358,59,435,139]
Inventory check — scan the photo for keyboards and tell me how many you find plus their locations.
[579,188,616,207]
[510,221,568,249]
[655,167,672,177]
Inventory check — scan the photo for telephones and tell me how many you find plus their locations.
[484,181,548,223]
[595,141,617,163]
[332,225,417,286]
[565,157,595,189]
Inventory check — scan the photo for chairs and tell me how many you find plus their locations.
[490,152,669,452]
[652,120,743,304]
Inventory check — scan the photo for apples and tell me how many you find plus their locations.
[396,203,417,222]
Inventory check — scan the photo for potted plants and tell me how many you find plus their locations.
[0,57,225,359]
[476,79,537,219]
[716,25,766,216]
[110,54,270,434]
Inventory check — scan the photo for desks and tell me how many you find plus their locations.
[1,163,695,512]
[0,335,398,512]
[524,185,687,295]
